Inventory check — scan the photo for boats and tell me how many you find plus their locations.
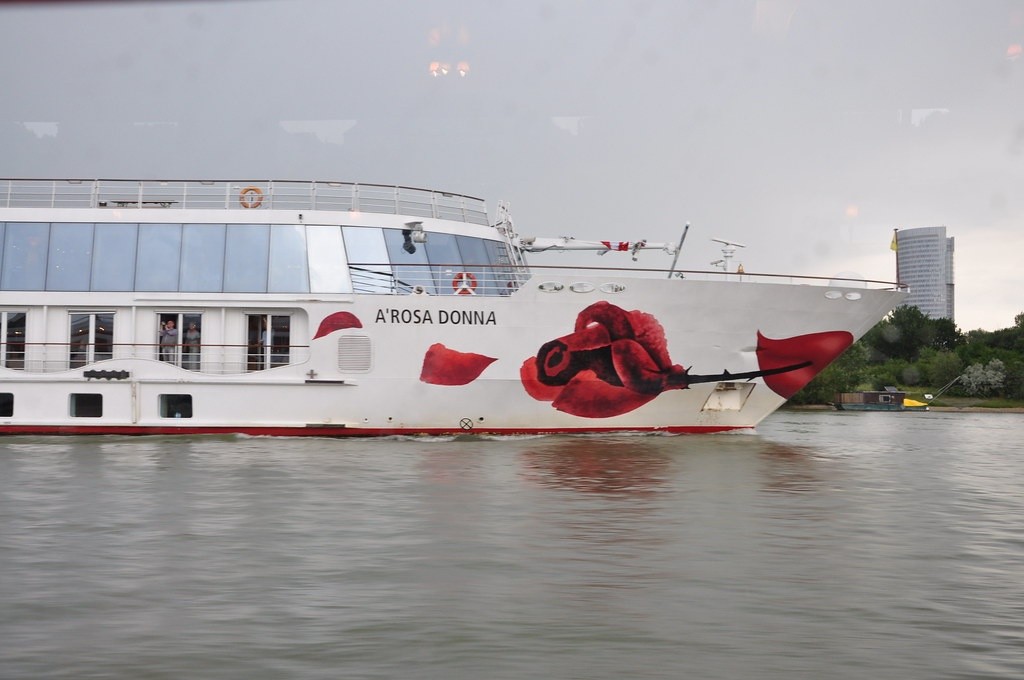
[0,178,912,437]
[841,391,928,413]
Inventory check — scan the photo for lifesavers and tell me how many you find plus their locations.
[239,186,263,208]
[452,271,477,294]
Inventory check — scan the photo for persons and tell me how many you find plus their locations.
[161,319,274,370]
